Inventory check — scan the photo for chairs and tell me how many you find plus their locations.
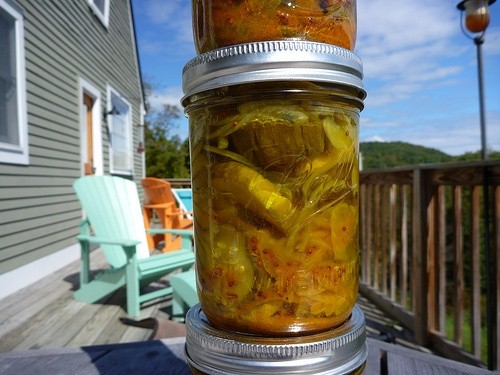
[74,175,201,322]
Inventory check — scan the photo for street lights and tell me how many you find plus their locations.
[456,0,500,371]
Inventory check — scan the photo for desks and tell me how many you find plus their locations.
[0,334,500,375]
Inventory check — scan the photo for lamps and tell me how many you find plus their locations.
[456,0,497,43]
[105,106,120,117]
